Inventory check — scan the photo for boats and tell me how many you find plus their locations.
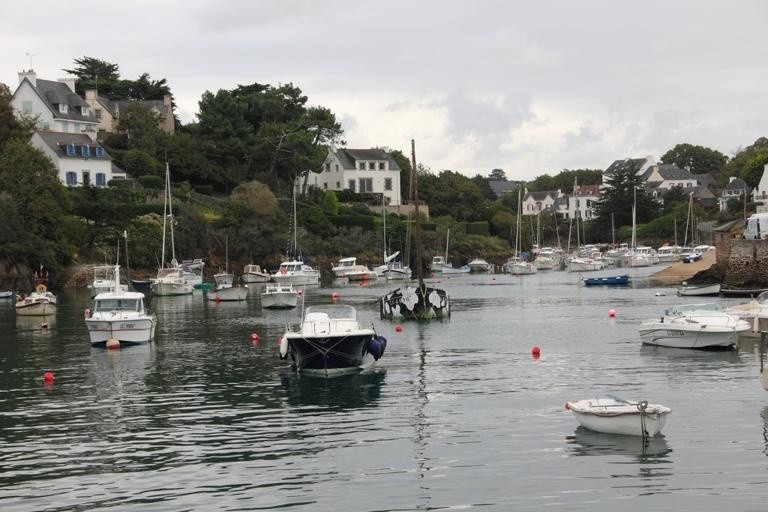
[678,280,722,297]
[262,304,300,327]
[330,250,377,280]
[0,290,13,298]
[429,256,453,272]
[87,249,129,300]
[0,297,12,306]
[88,340,157,419]
[241,264,270,289]
[281,367,386,420]
[640,339,741,367]
[565,397,671,438]
[83,239,158,348]
[572,425,669,460]
[468,258,490,272]
[16,310,57,339]
[639,303,751,349]
[16,290,56,316]
[207,298,247,318]
[723,291,767,338]
[204,283,249,301]
[279,304,387,379]
[577,273,631,287]
[260,283,298,309]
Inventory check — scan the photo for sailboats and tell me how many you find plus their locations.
[379,140,450,321]
[392,321,444,511]
[496,175,711,277]
[212,236,235,289]
[270,179,321,286]
[127,161,193,298]
[142,293,192,355]
[439,227,470,274]
[375,194,413,281]
